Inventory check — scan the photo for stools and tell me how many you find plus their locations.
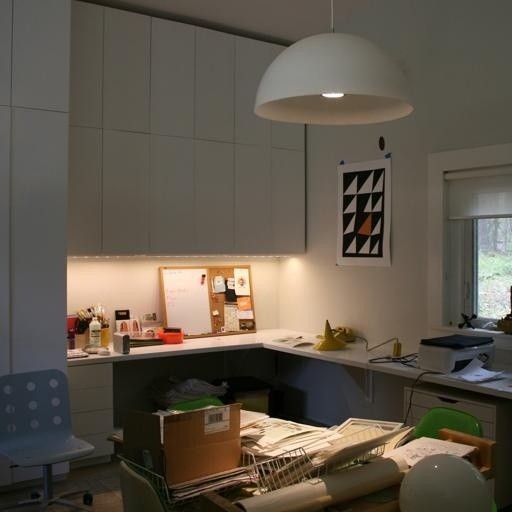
[215,376,273,414]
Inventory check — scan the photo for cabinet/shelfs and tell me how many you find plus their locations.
[72,4,308,258]
[403,385,512,510]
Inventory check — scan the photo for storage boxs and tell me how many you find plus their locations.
[123,402,241,486]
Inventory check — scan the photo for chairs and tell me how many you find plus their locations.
[120,461,164,512]
[0,369,95,512]
[409,407,497,512]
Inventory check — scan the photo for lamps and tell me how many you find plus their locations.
[253,0,415,126]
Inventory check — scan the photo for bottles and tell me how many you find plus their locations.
[88,315,102,347]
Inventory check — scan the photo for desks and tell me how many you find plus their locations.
[106,409,497,511]
[67,329,512,510]
